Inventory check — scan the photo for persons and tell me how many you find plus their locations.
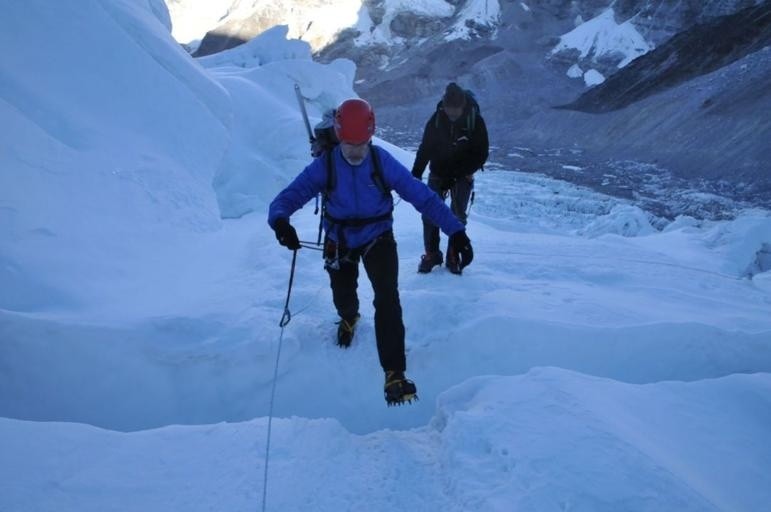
[268,97,476,409]
[410,82,490,275]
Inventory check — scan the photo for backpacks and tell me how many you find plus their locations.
[435,93,479,132]
[314,109,391,195]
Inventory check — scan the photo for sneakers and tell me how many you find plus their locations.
[418,250,443,271]
[337,315,358,345]
[447,251,462,272]
[383,369,417,404]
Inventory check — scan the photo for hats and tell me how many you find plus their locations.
[443,83,467,108]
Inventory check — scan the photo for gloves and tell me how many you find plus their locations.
[449,230,473,264]
[271,218,301,248]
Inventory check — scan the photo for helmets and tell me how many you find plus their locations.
[335,100,373,144]
